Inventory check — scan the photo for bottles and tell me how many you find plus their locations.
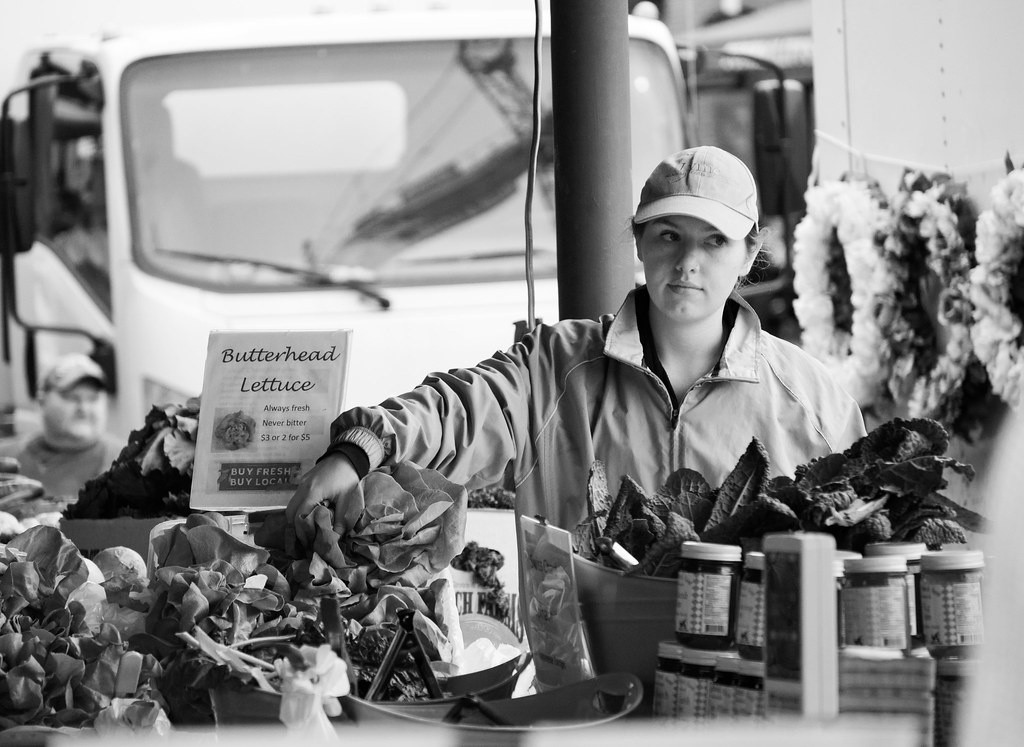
[651,536,987,717]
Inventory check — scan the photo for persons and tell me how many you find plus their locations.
[284,145,869,552]
[1,353,128,499]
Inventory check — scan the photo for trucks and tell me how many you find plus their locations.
[0,2,809,476]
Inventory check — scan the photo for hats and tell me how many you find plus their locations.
[44,354,110,394]
[633,145,759,240]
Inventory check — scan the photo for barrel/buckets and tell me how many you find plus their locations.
[0,507,673,747]
